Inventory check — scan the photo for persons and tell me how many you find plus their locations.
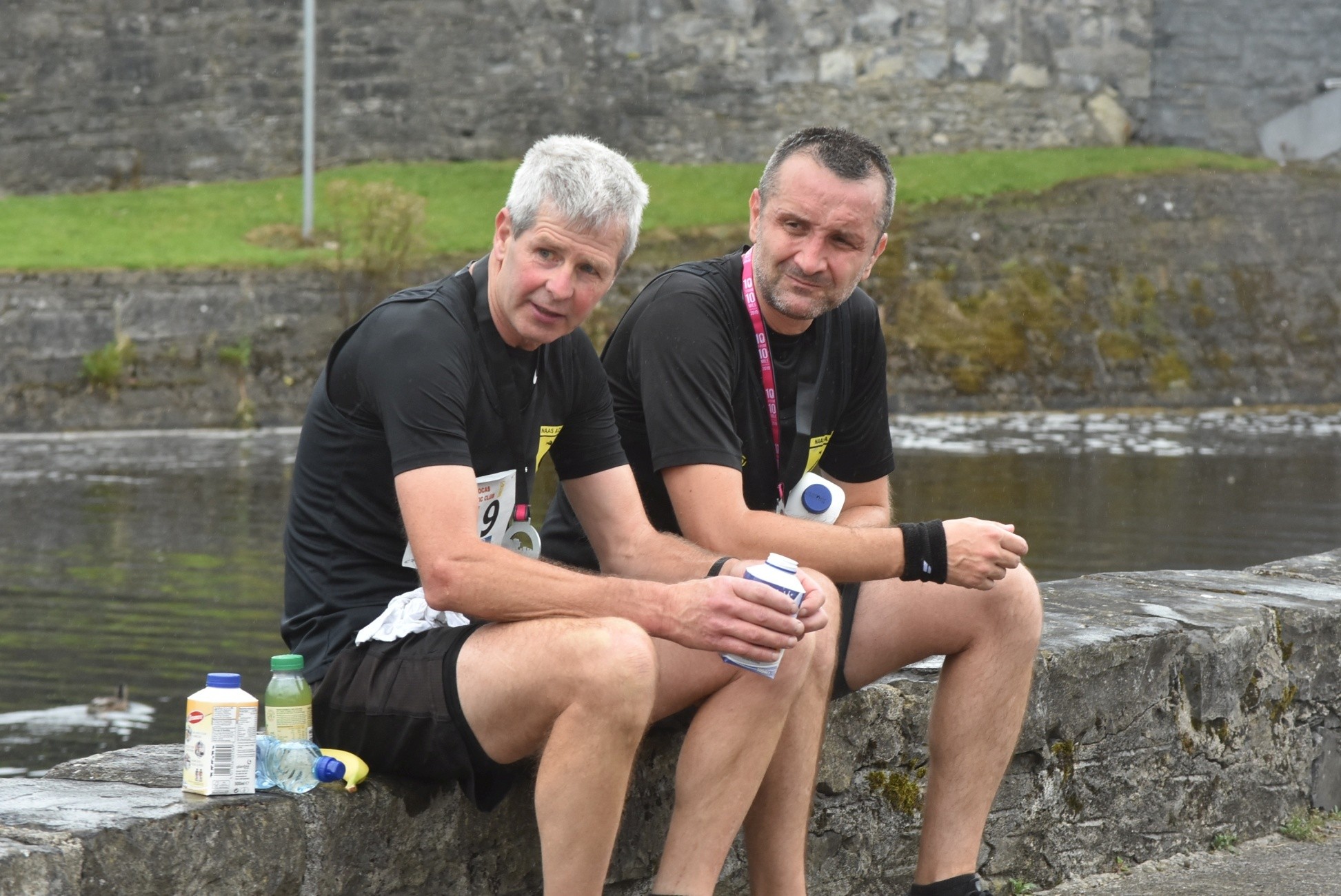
[280,134,829,896]
[538,127,1043,896]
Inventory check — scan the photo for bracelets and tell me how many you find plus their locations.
[899,519,947,585]
[706,557,738,576]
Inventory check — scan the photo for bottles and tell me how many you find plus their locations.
[257,734,345,793]
[779,471,849,527]
[721,553,805,678]
[183,674,259,795]
[265,654,314,743]
